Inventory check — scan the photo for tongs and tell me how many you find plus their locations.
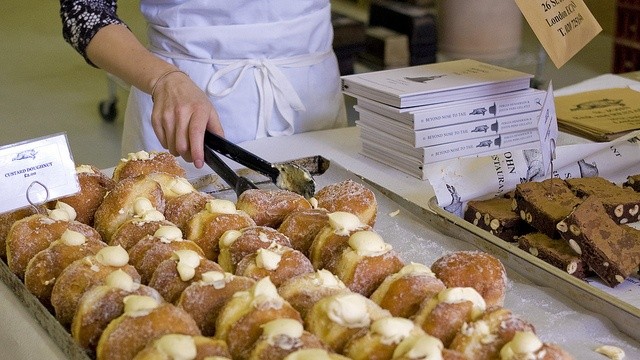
[203,129,316,200]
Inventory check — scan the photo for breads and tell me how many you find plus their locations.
[463,173,639,288]
[1,149,576,359]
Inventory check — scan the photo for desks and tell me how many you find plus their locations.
[0,72,636,359]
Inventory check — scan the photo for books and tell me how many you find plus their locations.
[338,57,548,181]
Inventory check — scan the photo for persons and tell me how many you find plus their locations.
[57,0,348,168]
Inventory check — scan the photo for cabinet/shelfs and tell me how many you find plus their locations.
[613,0,640,73]
